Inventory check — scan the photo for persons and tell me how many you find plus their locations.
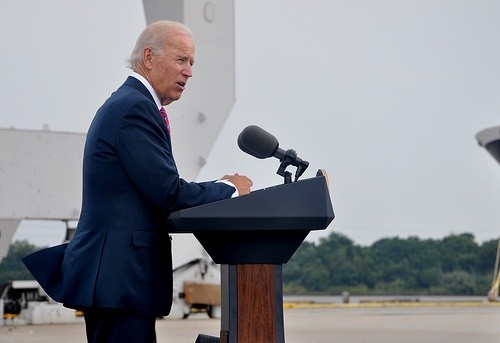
[22,20,254,343]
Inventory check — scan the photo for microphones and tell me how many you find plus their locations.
[237,125,309,177]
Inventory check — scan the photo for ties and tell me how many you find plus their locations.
[160,107,171,137]
